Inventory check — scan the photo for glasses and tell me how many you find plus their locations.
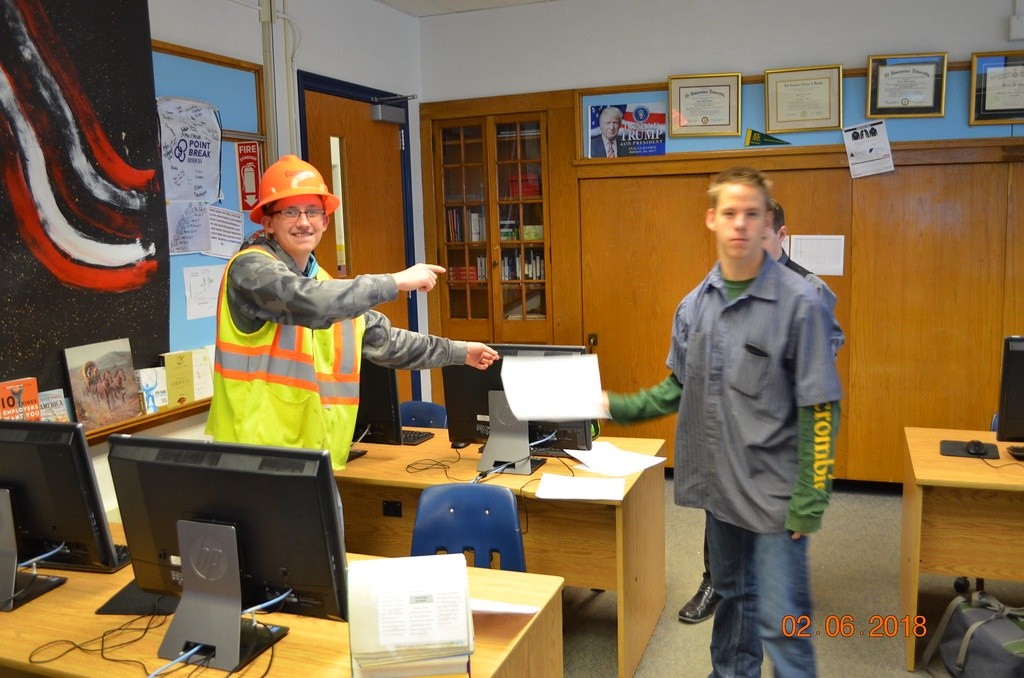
[264,208,325,223]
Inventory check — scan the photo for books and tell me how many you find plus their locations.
[445,175,546,281]
[349,554,474,678]
[0,338,143,433]
[137,344,215,414]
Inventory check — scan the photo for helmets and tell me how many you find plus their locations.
[250,155,340,224]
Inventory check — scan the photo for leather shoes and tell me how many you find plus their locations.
[679,579,721,623]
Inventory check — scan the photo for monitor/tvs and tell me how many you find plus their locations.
[0,419,118,611]
[442,343,593,476]
[346,358,403,463]
[106,432,349,672]
[996,335,1024,442]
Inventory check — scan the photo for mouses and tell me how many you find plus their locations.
[967,440,986,455]
[451,442,470,448]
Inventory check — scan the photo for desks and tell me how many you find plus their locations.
[902,427,1024,671]
[0,522,564,677]
[335,427,666,678]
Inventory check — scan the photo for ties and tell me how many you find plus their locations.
[608,140,615,158]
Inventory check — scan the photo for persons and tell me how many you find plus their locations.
[603,167,841,678]
[591,107,630,156]
[204,155,500,467]
[677,198,844,621]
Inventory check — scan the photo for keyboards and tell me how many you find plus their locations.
[20,544,132,574]
[1007,444,1024,461]
[478,444,573,458]
[402,429,435,445]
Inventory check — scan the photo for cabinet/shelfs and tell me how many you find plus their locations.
[431,109,555,347]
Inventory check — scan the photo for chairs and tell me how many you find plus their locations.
[399,401,449,428]
[409,483,526,572]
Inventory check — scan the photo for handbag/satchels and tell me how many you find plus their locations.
[923,594,1024,678]
